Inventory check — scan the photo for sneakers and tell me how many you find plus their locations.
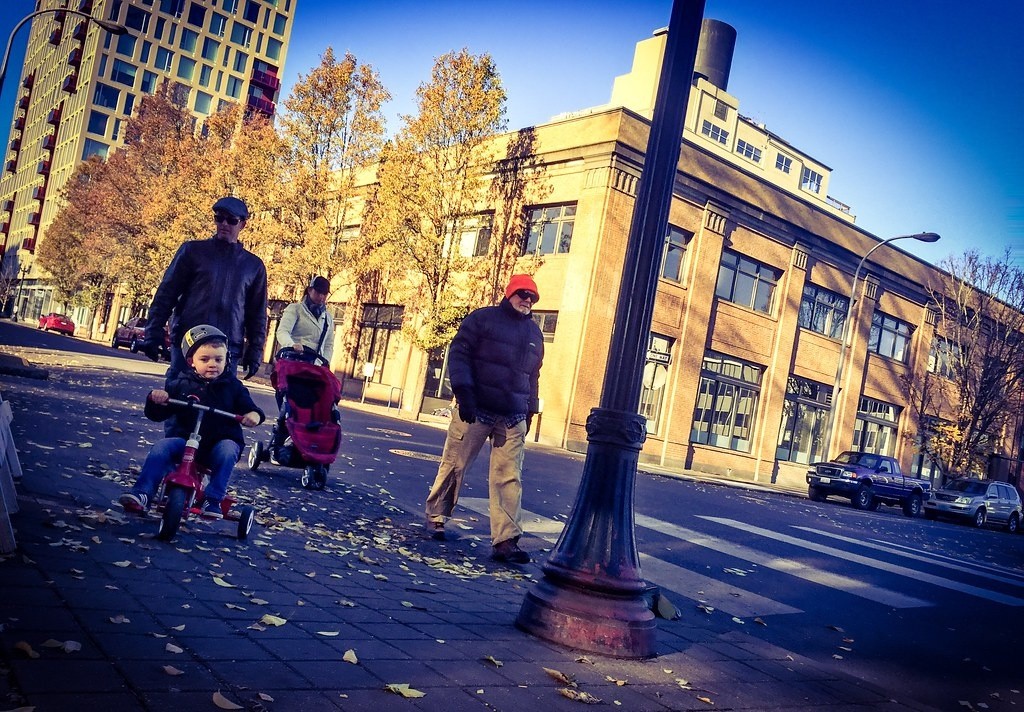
[425,515,446,538]
[493,539,530,563]
[119,492,152,512]
[201,498,224,519]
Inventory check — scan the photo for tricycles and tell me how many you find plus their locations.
[124,394,257,544]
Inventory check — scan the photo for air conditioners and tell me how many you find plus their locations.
[23,74,33,90]
[62,75,77,94]
[72,21,88,41]
[19,96,29,110]
[32,186,45,200]
[47,109,61,125]
[42,135,56,150]
[10,138,21,151]
[28,212,40,225]
[14,117,25,130]
[6,160,16,173]
[3,200,14,213]
[49,28,62,45]
[22,239,36,250]
[54,5,66,23]
[37,161,50,175]
[78,0,93,15]
[0,222,9,233]
[68,48,83,66]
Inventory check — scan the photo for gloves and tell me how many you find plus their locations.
[242,358,260,380]
[459,405,477,424]
[144,338,168,362]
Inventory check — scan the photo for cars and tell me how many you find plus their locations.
[38,312,75,337]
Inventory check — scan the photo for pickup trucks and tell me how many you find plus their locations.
[111,316,172,362]
[806,450,936,518]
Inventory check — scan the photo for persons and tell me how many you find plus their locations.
[143,197,267,436]
[120,325,266,519]
[270,277,335,465]
[424,273,545,562]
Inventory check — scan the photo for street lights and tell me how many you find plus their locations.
[11,258,34,323]
[821,230,942,463]
[1,7,131,99]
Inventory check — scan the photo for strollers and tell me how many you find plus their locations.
[248,345,343,491]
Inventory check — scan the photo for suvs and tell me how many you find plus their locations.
[924,475,1024,534]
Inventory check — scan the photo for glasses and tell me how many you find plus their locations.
[214,215,238,225]
[517,290,537,302]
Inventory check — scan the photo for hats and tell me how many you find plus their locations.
[505,274,540,302]
[212,197,249,217]
[309,276,331,294]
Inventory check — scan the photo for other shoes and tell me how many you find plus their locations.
[270,451,282,466]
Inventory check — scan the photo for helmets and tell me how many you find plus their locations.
[181,324,229,360]
